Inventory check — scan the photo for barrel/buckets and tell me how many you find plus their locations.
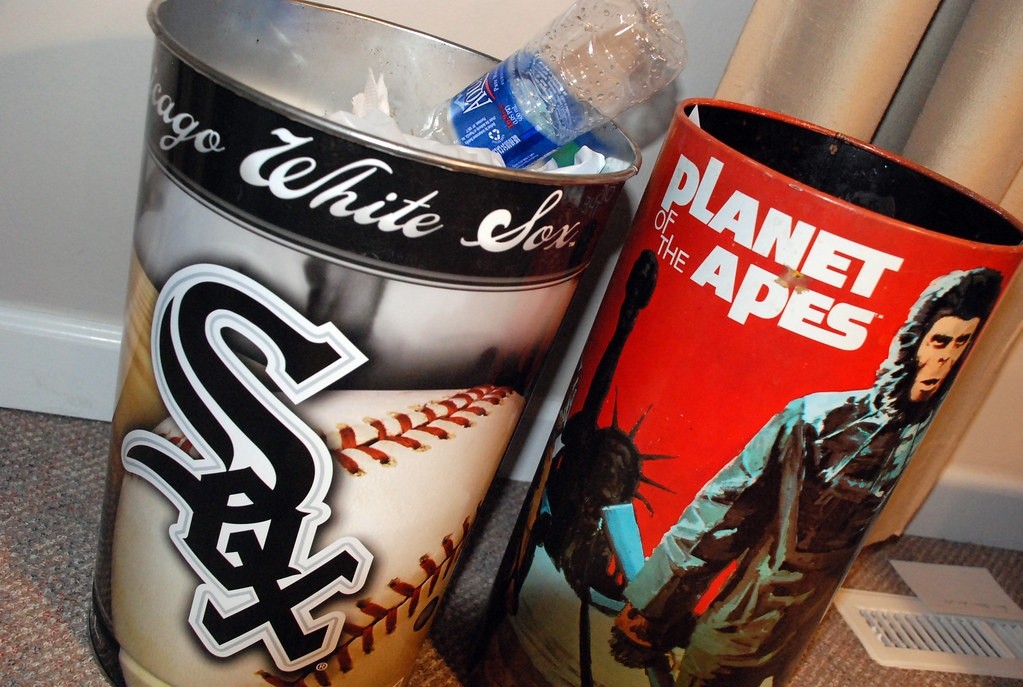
[87,0,642,686]
[466,102,1022,687]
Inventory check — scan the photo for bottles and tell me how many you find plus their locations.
[418,1,686,171]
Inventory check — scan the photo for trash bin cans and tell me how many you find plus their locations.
[90,0,644,687]
[485,98,1021,687]
